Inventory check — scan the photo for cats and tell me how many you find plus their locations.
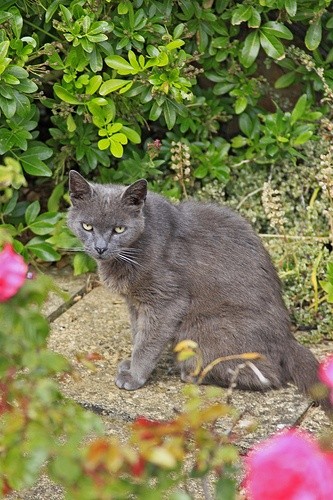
[65,170,333,422]
